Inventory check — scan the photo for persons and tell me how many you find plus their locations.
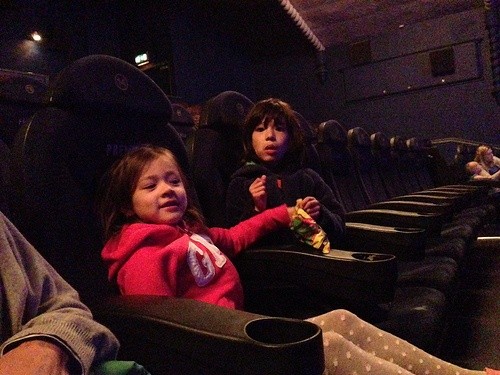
[0,208,119,375]
[101,144,500,375]
[226,97,347,249]
[465,145,500,182]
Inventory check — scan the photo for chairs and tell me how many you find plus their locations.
[0,54,500,375]
